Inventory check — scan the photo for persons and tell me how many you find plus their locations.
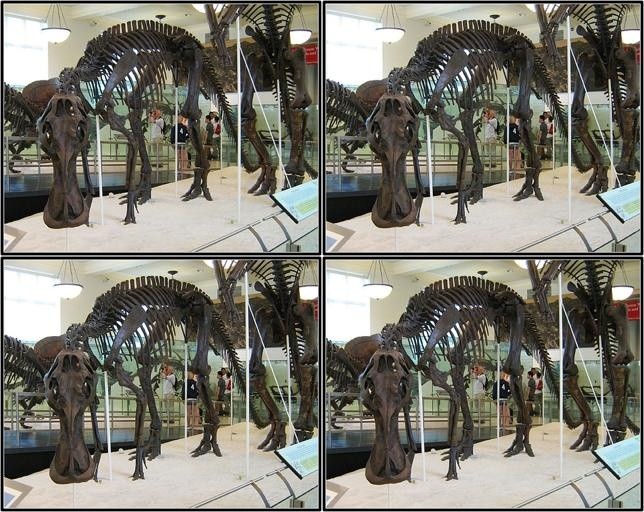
[504,116,525,169]
[482,111,497,168]
[472,367,487,424]
[160,366,177,423]
[215,368,232,416]
[181,372,202,426]
[538,111,556,160]
[147,109,164,167]
[170,116,192,169]
[204,112,220,160]
[526,367,543,417]
[492,373,513,425]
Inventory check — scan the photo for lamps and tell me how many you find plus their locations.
[288,4,312,46]
[38,3,70,45]
[52,260,83,302]
[295,258,318,302]
[616,5,641,46]
[611,258,636,303]
[361,259,393,303]
[374,5,406,45]
